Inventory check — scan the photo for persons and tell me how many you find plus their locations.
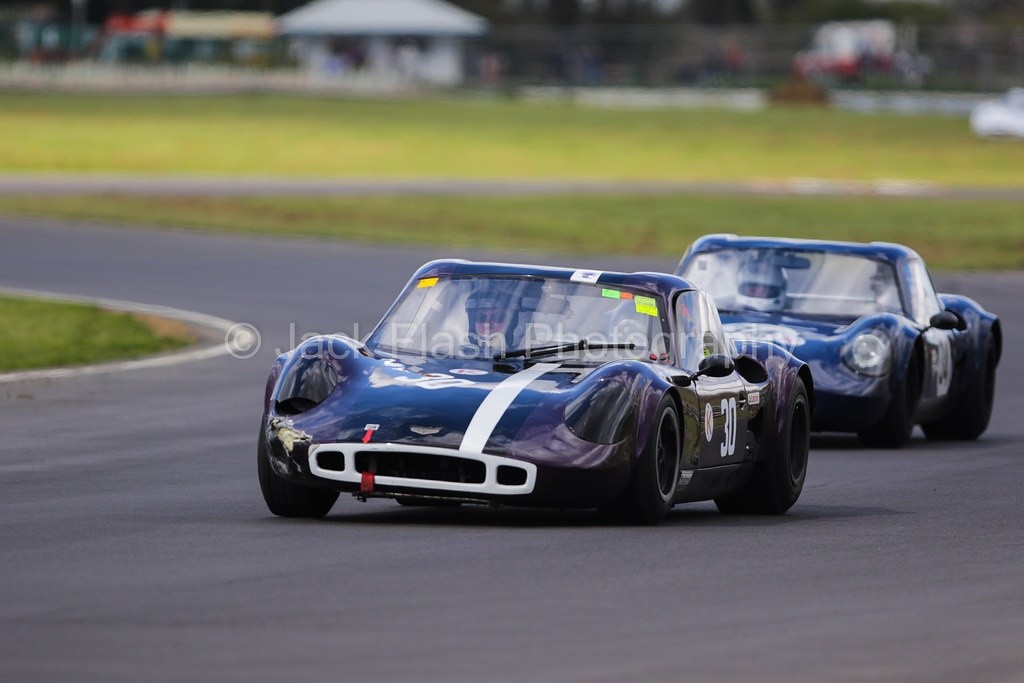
[465,286,529,361]
[732,261,789,313]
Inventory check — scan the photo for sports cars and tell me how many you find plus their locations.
[255,257,816,532]
[608,231,1005,451]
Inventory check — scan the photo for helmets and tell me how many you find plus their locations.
[465,284,522,350]
[734,261,788,314]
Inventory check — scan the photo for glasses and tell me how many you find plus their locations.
[471,311,507,323]
